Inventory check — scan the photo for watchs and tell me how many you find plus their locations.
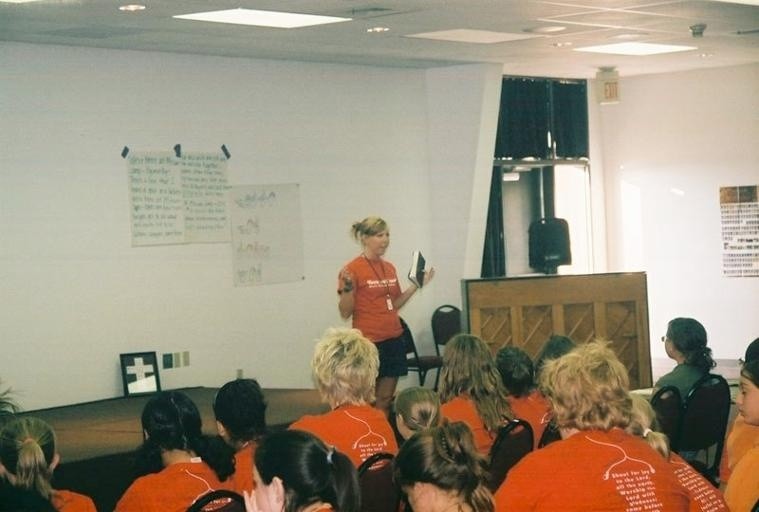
[342,287,354,293]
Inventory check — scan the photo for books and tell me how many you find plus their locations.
[408,250,426,288]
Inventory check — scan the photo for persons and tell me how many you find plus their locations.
[338,215,436,419]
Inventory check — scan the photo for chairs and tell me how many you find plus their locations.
[432,304,463,390]
[182,372,735,512]
[399,313,445,387]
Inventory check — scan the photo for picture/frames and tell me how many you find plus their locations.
[120,349,162,397]
